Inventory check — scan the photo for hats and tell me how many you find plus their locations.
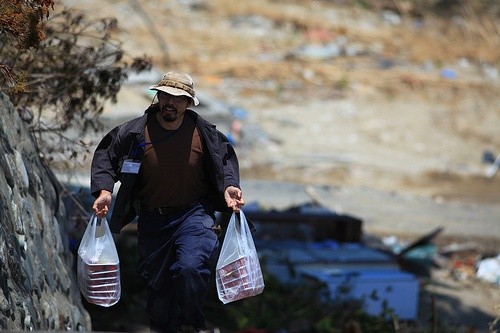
[149,71,199,106]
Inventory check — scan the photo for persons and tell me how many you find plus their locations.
[89,72,256,333]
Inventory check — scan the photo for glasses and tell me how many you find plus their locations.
[158,92,189,103]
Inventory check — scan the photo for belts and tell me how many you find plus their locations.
[140,207,189,216]
